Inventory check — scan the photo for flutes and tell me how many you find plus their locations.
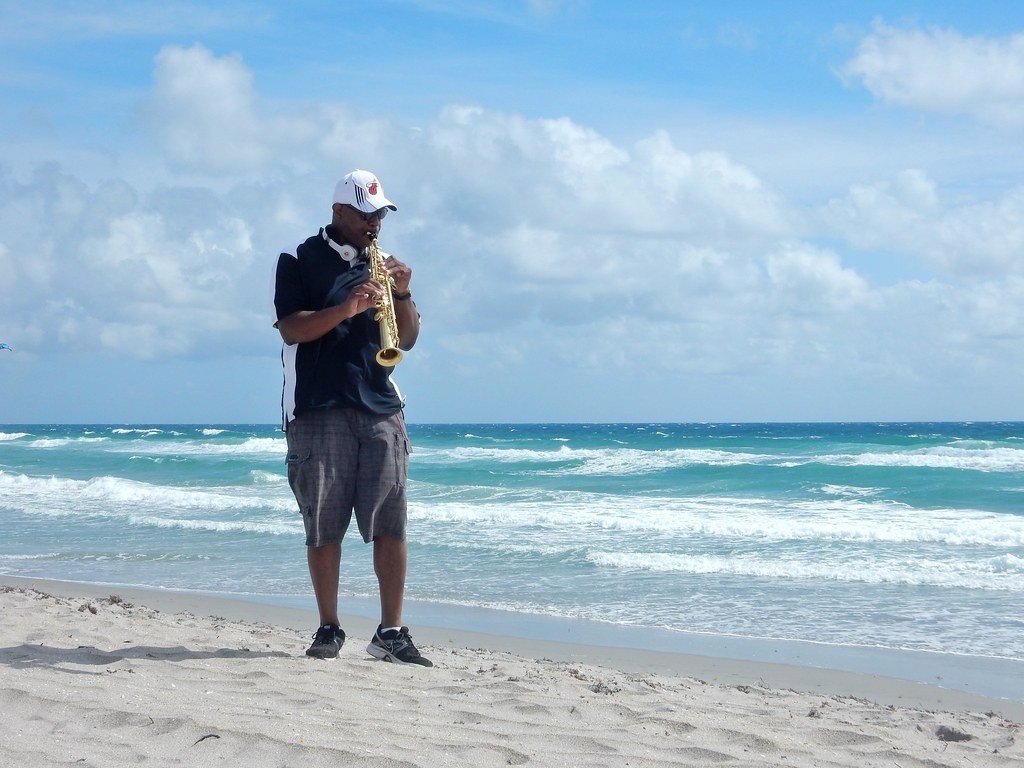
[365,230,404,368]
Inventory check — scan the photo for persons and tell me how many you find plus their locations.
[268,169,434,669]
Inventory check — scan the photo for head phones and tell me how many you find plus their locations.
[321,223,369,261]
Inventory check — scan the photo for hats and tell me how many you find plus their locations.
[332,168,399,213]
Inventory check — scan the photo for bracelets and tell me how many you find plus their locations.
[393,288,411,300]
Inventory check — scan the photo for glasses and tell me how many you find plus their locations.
[351,205,388,219]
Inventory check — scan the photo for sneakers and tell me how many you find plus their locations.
[366,624,433,668]
[306,623,346,660]
